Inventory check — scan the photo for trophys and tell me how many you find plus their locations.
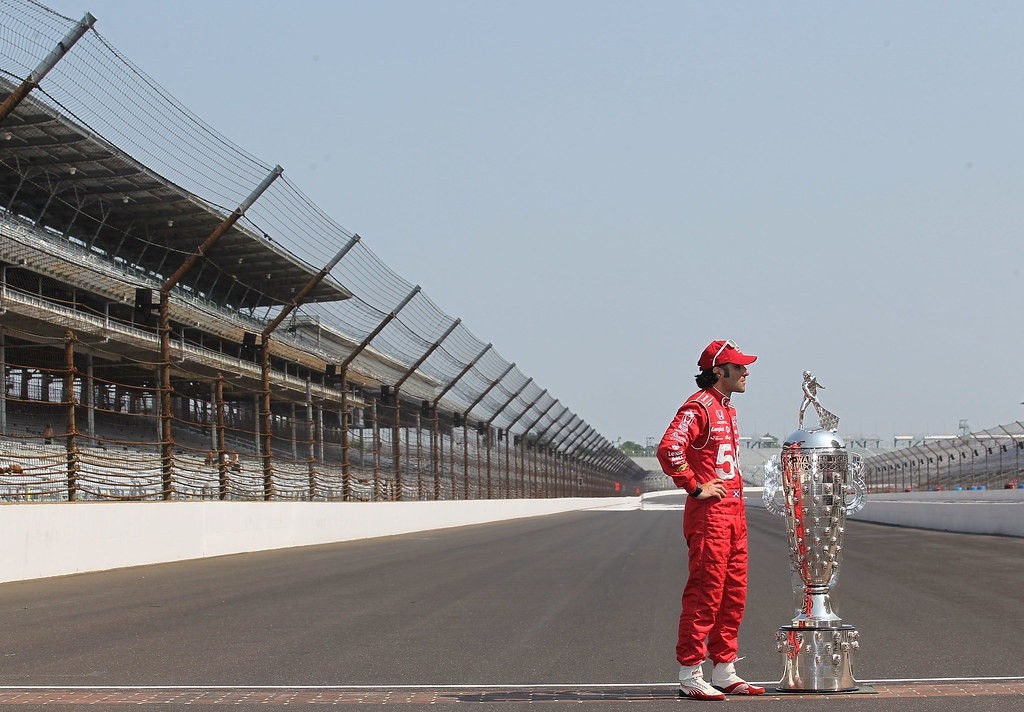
[762,369,868,696]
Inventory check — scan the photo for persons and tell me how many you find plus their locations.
[797,371,826,430]
[657,338,766,701]
[207,445,242,475]
[43,423,54,444]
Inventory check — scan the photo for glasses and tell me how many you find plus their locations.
[712,339,739,366]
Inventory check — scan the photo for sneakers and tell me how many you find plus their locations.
[679,661,725,701]
[711,656,765,695]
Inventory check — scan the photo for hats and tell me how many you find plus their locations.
[698,340,757,371]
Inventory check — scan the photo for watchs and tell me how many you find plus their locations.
[689,482,704,498]
